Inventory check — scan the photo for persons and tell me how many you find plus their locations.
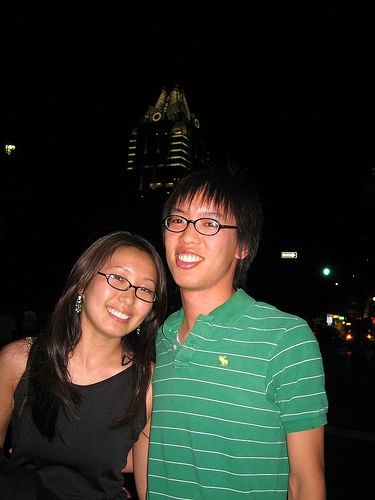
[0,231,166,500]
[132,168,329,500]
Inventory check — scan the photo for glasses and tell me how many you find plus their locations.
[98,272,158,303]
[162,214,241,236]
